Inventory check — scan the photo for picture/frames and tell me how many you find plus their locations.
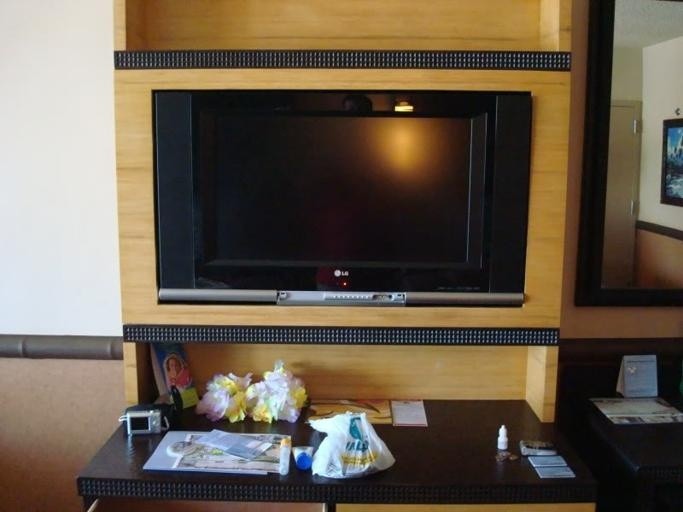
[660,118,683,207]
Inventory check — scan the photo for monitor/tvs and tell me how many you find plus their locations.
[150,89,534,308]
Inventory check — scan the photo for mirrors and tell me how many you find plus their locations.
[573,0,683,308]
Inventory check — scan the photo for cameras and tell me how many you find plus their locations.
[124,405,161,436]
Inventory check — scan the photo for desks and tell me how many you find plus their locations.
[74,399,597,512]
[561,386,683,512]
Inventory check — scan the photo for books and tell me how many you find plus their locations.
[303,395,393,427]
[390,397,428,428]
[149,342,200,412]
[590,394,682,427]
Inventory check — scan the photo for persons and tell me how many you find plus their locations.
[162,353,191,400]
